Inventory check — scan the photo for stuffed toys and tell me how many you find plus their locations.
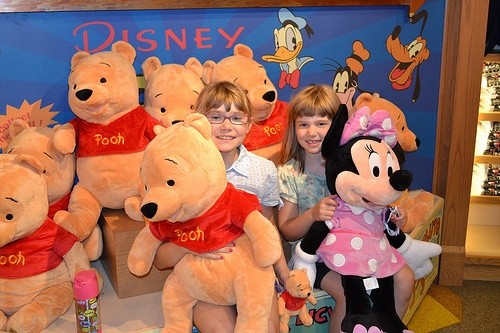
[0,154,103,333]
[53,41,167,242]
[126,113,281,333]
[141,57,204,126]
[293,105,441,333]
[350,93,434,232]
[277,268,317,333]
[202,44,290,165]
[5,118,103,261]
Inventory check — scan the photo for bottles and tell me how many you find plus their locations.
[73,270,102,333]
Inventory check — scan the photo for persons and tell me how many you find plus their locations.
[277,85,414,333]
[154,81,279,332]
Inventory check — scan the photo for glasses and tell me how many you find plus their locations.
[206,112,250,126]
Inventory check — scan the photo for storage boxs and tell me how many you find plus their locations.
[100,210,174,299]
[389,189,444,325]
